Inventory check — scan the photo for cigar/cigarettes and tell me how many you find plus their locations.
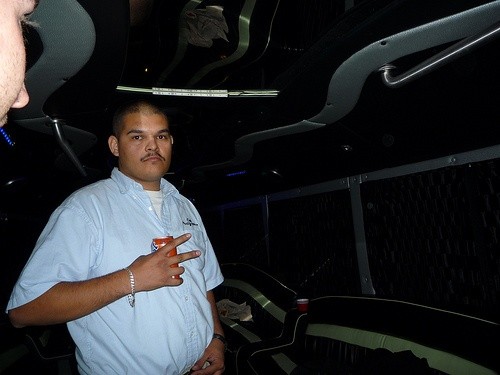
[0,127,15,146]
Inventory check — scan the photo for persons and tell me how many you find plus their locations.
[0,0,41,128]
[6,100,226,375]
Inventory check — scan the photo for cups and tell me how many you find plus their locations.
[296,299,309,312]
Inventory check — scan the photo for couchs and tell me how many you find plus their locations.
[24,262,500,375]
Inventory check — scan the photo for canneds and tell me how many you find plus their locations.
[151,235,179,279]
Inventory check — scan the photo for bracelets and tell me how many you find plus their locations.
[125,268,135,307]
[213,334,225,344]
